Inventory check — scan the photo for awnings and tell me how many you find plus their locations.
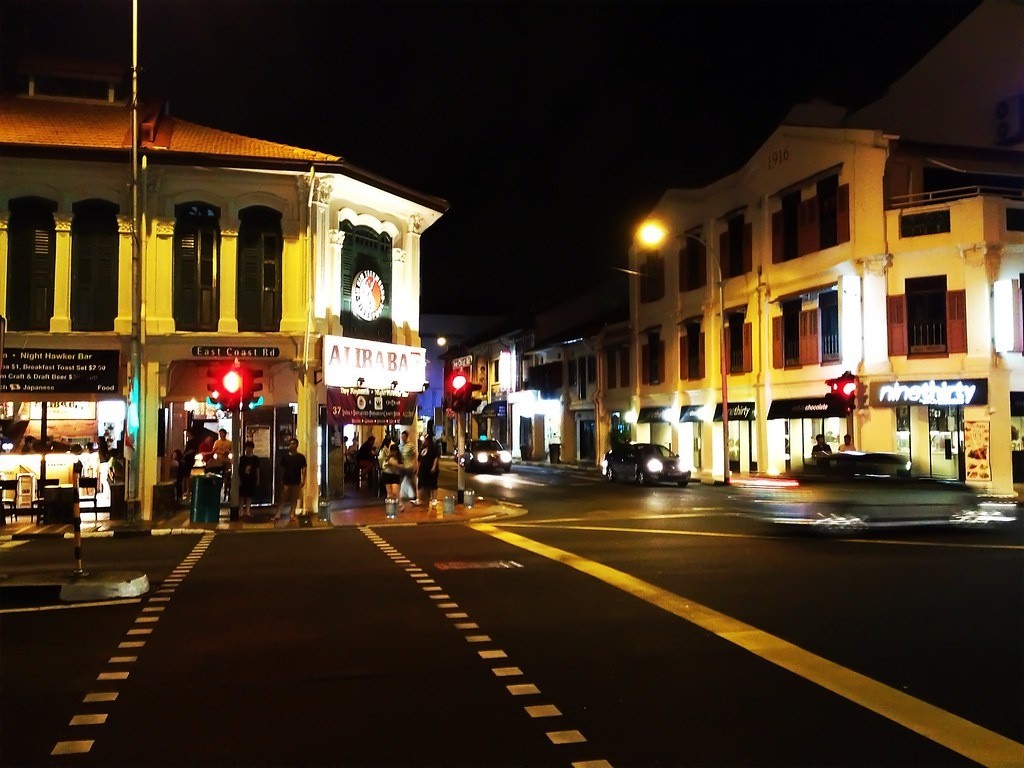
[767,397,846,421]
[925,156,1024,178]
[637,407,671,426]
[714,402,756,422]
[679,405,704,423]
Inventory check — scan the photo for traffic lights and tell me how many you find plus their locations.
[221,370,245,395]
[451,375,467,413]
[842,382,858,397]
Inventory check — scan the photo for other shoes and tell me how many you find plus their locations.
[432,499,437,506]
[410,500,420,506]
[399,501,405,512]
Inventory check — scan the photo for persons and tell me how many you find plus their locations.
[441,431,448,455]
[839,435,856,452]
[344,436,376,485]
[377,431,439,505]
[812,434,831,458]
[170,428,231,502]
[239,442,261,517]
[274,438,307,520]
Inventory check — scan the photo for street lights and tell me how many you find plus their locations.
[635,220,731,486]
[238,366,265,410]
[205,368,231,408]
[467,382,482,415]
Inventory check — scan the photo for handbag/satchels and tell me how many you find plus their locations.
[400,477,414,500]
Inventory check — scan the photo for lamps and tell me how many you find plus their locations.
[357,377,430,393]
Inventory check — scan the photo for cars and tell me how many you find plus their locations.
[605,448,691,488]
[453,437,512,473]
[735,449,981,535]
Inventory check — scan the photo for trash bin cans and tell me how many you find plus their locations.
[43,485,74,523]
[190,475,222,522]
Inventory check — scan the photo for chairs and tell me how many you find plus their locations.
[0,477,97,525]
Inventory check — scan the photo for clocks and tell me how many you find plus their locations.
[352,269,385,322]
